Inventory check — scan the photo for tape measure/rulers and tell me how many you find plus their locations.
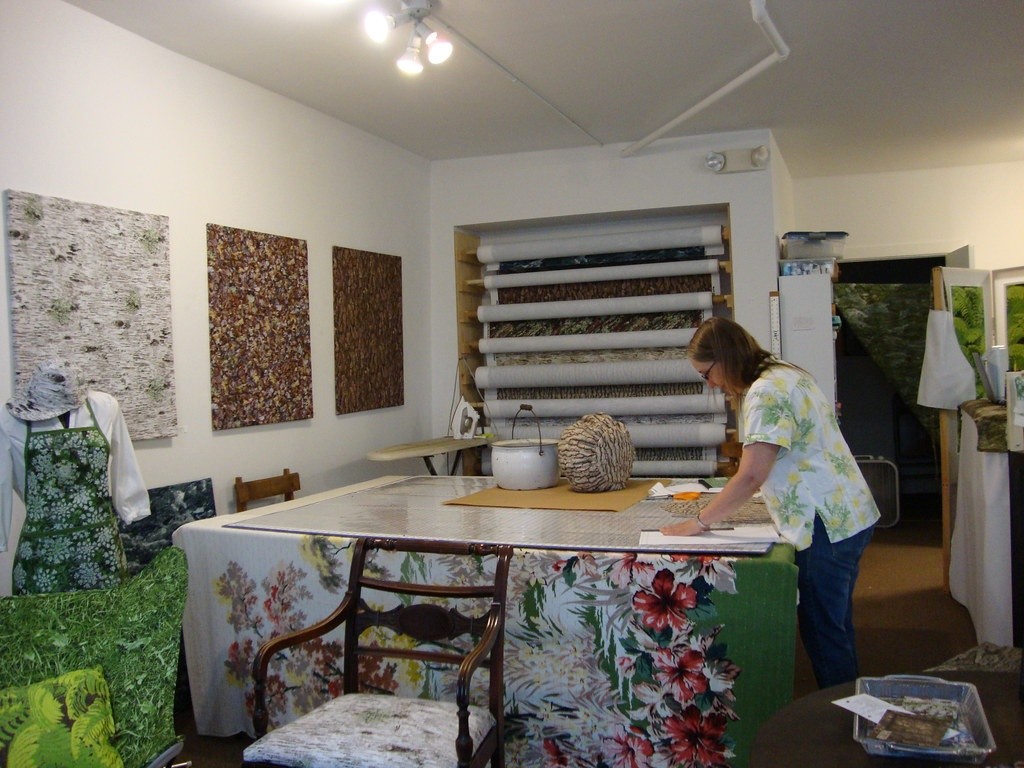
[642,528,736,533]
[768,290,783,364]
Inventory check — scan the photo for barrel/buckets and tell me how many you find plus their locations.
[490,439,559,490]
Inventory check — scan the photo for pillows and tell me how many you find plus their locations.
[0,544,191,767]
[0,663,124,768]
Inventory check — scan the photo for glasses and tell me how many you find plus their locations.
[701,360,718,381]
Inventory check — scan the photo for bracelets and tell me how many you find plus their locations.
[696,511,707,528]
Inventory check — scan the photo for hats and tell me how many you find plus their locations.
[5,356,88,421]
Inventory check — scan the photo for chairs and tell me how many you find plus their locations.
[233,468,301,514]
[242,537,513,768]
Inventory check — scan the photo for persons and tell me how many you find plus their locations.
[659,317,881,690]
[0,357,152,594]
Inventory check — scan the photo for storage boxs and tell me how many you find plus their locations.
[781,230,850,260]
[848,673,997,767]
[780,256,840,282]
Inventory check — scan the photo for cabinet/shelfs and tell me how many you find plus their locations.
[776,274,841,426]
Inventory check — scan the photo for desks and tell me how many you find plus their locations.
[168,474,800,768]
[366,435,489,477]
